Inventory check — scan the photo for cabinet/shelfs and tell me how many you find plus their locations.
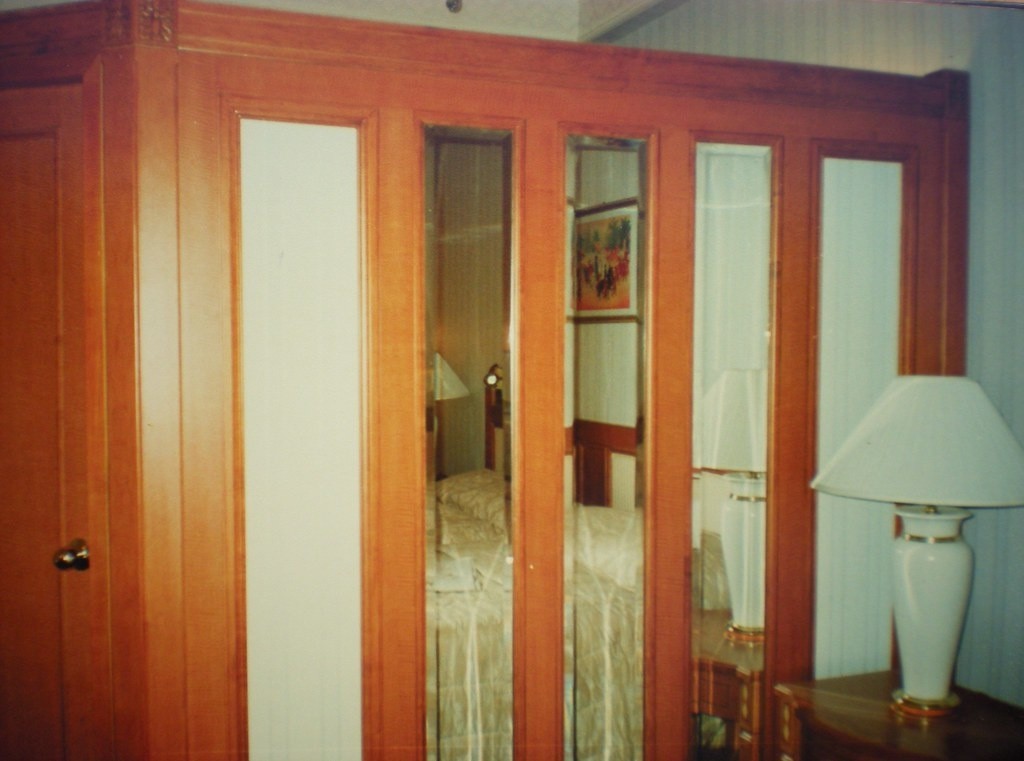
[144,4,973,761]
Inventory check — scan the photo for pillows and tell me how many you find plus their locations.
[436,468,513,537]
[576,502,645,594]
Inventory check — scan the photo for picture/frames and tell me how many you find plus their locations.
[571,195,638,321]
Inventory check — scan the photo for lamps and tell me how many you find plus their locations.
[693,370,768,646]
[812,376,1023,729]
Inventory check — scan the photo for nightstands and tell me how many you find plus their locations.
[778,670,1024,761]
[688,621,764,761]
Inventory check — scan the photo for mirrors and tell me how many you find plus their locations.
[241,117,907,761]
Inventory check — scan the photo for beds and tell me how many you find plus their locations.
[427,367,729,761]
